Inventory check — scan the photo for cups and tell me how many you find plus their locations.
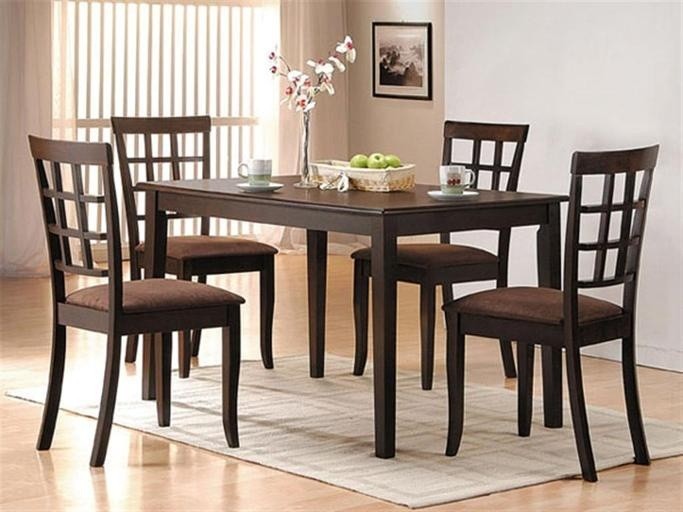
[438,165,476,194]
[236,158,272,184]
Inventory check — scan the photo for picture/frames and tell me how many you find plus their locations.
[371,20,433,100]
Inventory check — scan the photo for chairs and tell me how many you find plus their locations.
[29,134,242,469]
[440,143,661,486]
[350,118,531,390]
[111,116,278,380]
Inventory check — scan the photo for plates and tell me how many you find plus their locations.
[234,182,285,192]
[428,191,480,202]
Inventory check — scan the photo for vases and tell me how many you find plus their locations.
[293,112,317,188]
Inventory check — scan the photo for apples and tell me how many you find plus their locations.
[350,153,400,169]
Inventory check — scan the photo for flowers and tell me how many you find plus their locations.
[268,34,357,175]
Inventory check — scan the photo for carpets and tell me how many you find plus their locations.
[5,351,683,511]
